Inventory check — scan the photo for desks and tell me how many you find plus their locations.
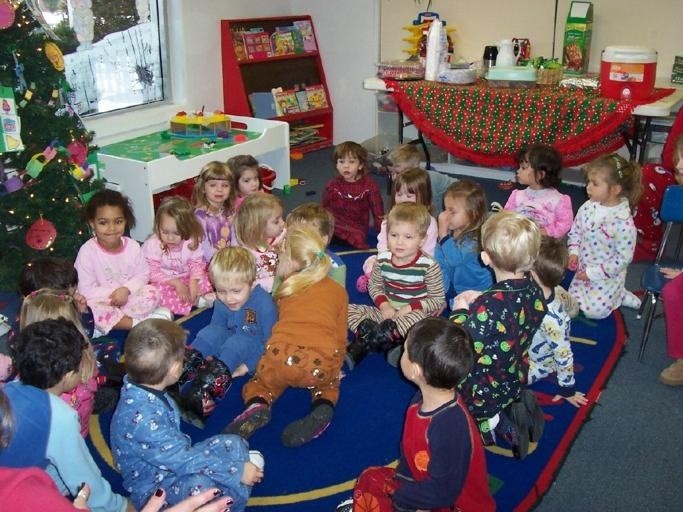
[96,112,289,243]
[364,74,682,171]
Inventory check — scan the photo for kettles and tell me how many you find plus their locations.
[496,39,522,67]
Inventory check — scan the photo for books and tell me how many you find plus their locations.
[232,20,331,149]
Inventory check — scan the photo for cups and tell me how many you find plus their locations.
[423,19,445,82]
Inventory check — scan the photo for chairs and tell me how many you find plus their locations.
[636,182,683,363]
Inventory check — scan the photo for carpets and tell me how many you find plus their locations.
[0,226,628,511]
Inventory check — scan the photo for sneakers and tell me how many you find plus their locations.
[622,288,642,310]
[282,403,334,448]
[659,358,683,386]
[496,389,546,460]
[222,403,272,439]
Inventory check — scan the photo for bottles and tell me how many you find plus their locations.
[483,45,498,69]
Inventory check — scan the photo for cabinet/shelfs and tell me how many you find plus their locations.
[219,16,333,153]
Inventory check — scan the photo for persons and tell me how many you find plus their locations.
[0,143,644,512]
[657,133,683,390]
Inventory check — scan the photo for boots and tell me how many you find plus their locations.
[169,348,233,419]
[345,318,404,371]
